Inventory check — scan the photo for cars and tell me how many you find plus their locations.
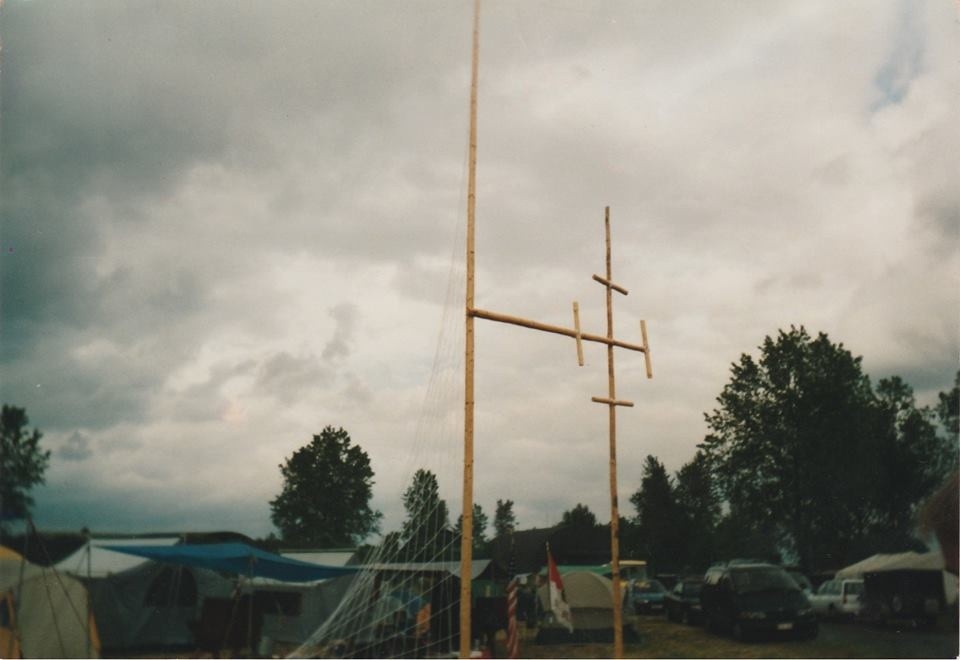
[622,560,816,640]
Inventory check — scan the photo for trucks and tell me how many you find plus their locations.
[858,567,945,630]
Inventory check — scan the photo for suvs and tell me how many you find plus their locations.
[807,578,868,625]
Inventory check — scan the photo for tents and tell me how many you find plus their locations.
[833,550,960,624]
[1,527,509,660]
[536,560,651,632]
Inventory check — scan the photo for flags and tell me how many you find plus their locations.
[546,542,574,635]
